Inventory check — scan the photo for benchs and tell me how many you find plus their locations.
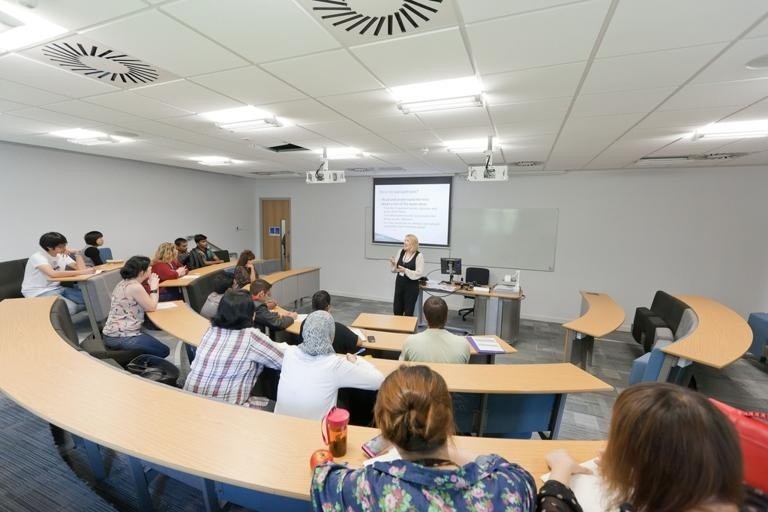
[748,313,768,364]
[72,248,113,290]
[0,258,73,300]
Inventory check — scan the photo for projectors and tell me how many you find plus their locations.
[306,171,346,183]
[468,165,508,181]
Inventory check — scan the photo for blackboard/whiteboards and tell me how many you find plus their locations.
[364,206,559,272]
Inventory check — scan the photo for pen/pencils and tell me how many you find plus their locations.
[354,348,366,355]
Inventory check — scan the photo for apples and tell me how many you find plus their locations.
[310,449,333,469]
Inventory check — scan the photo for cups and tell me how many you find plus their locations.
[320,405,350,458]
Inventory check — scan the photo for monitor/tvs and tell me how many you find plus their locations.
[441,258,461,285]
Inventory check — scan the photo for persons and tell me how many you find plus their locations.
[387,234,426,316]
[399,296,471,364]
[21,233,86,315]
[198,250,297,334]
[79,231,103,267]
[184,291,284,405]
[538,382,745,512]
[104,255,171,366]
[300,290,362,353]
[143,234,224,302]
[275,310,384,418]
[310,365,538,512]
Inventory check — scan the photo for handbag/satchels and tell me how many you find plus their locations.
[128,354,180,385]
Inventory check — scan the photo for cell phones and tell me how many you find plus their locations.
[368,336,375,342]
[361,432,394,458]
